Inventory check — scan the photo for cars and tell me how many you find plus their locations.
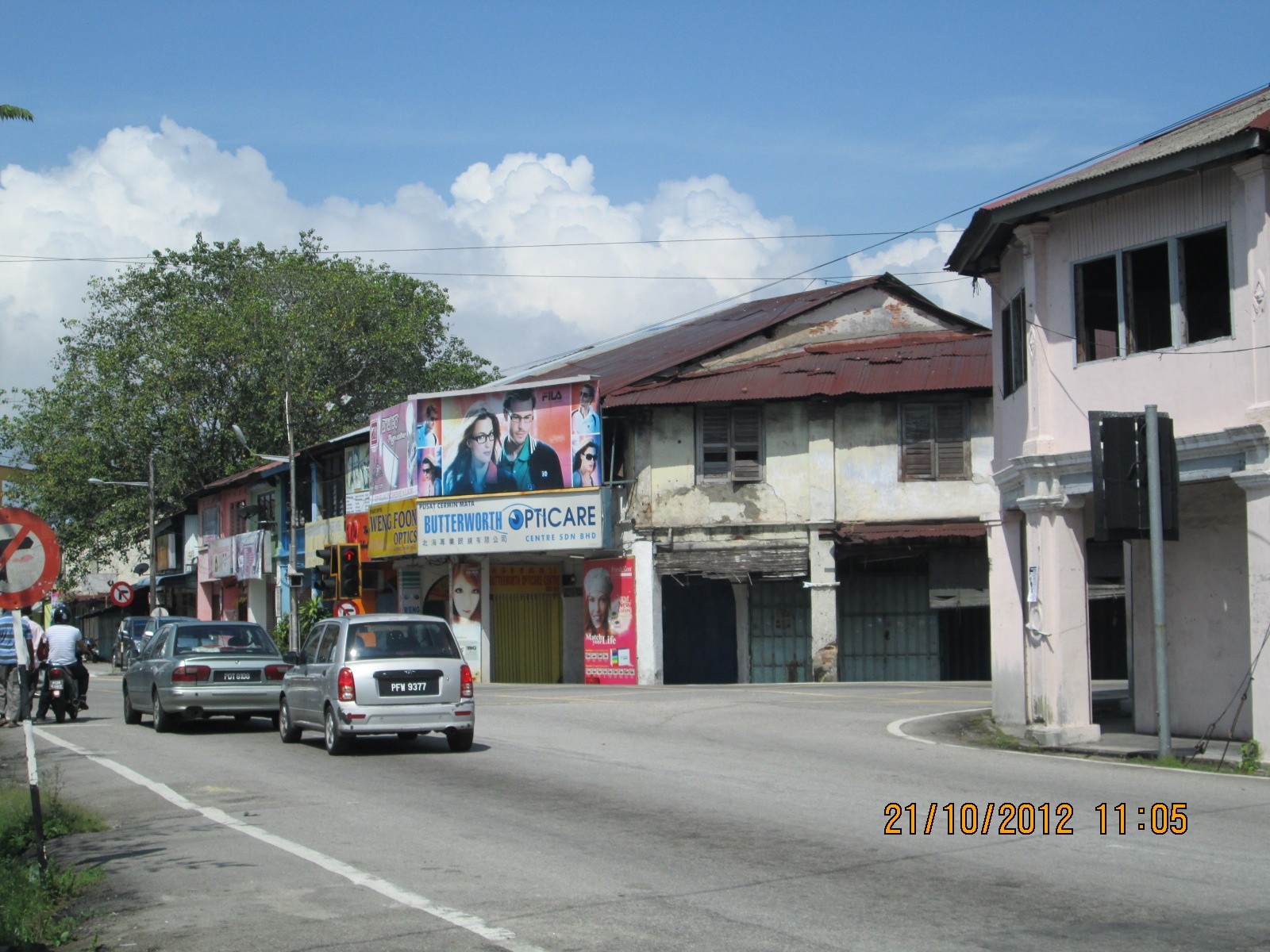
[112,616,153,671]
[279,613,475,756]
[140,616,218,658]
[123,620,295,733]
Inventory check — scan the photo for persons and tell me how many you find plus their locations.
[584,567,613,640]
[0,606,89,727]
[573,383,602,488]
[80,635,95,662]
[452,561,482,636]
[442,388,564,497]
[813,636,838,682]
[346,452,369,494]
[417,406,441,496]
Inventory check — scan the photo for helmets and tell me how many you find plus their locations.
[53,607,71,622]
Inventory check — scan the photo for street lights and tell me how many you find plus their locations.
[86,452,157,618]
[231,413,300,660]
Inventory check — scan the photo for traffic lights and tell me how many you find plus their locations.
[315,545,338,602]
[337,543,362,600]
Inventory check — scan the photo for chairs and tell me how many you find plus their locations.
[387,630,407,654]
[228,637,247,646]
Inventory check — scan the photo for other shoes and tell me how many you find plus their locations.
[7,723,20,728]
[0,718,7,727]
[79,700,89,710]
[33,718,45,724]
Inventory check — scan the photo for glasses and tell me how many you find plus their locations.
[580,391,590,401]
[428,415,438,423]
[471,431,495,444]
[506,410,532,422]
[423,467,431,474]
[583,453,598,460]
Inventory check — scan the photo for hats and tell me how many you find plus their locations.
[584,568,613,595]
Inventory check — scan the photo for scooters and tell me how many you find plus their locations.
[43,651,88,724]
